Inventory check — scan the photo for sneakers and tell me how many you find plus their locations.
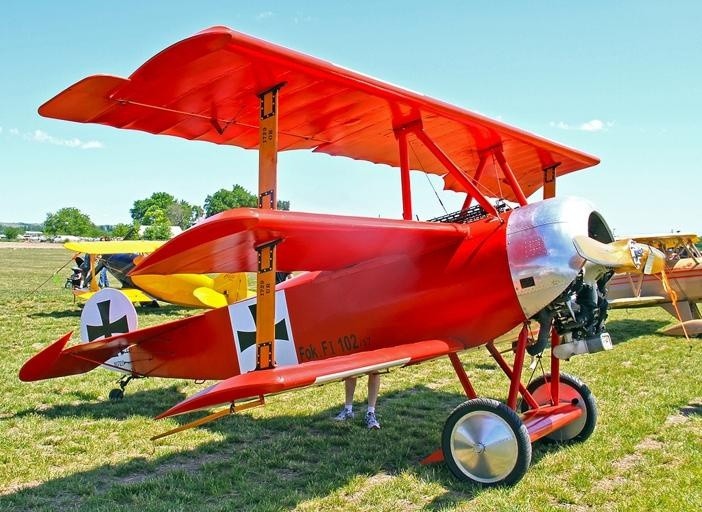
[335,408,353,420]
[365,411,380,429]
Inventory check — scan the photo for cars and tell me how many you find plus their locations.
[0,231,125,244]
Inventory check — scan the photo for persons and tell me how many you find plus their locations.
[332,372,381,430]
[98,236,110,288]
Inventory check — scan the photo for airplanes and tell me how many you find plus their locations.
[63,229,258,309]
[19,25,665,490]
[604,232,701,338]
